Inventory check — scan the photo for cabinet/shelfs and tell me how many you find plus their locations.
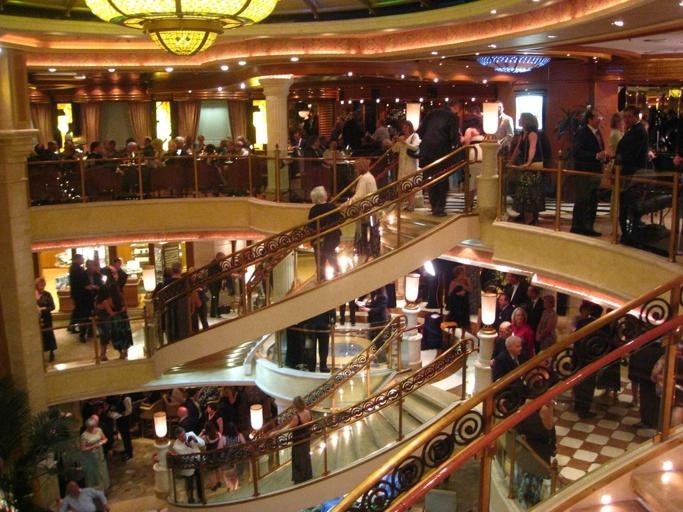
[129,246,149,265]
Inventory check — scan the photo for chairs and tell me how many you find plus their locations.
[140,399,162,441]
[162,394,182,440]
[129,397,148,427]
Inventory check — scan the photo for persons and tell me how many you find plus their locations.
[36,252,226,365]
[568,103,680,235]
[59,309,337,510]
[507,113,545,226]
[289,101,515,257]
[29,135,251,206]
[340,265,472,352]
[350,158,380,264]
[484,273,662,509]
[308,185,343,281]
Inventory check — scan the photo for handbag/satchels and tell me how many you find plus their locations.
[523,162,543,169]
[407,146,420,157]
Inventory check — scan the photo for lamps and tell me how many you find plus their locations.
[142,265,156,299]
[482,102,498,143]
[477,54,551,73]
[85,0,278,56]
[480,294,497,335]
[404,273,420,309]
[406,103,420,131]
[153,412,169,445]
[250,404,264,436]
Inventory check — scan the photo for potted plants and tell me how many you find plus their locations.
[551,105,585,203]
